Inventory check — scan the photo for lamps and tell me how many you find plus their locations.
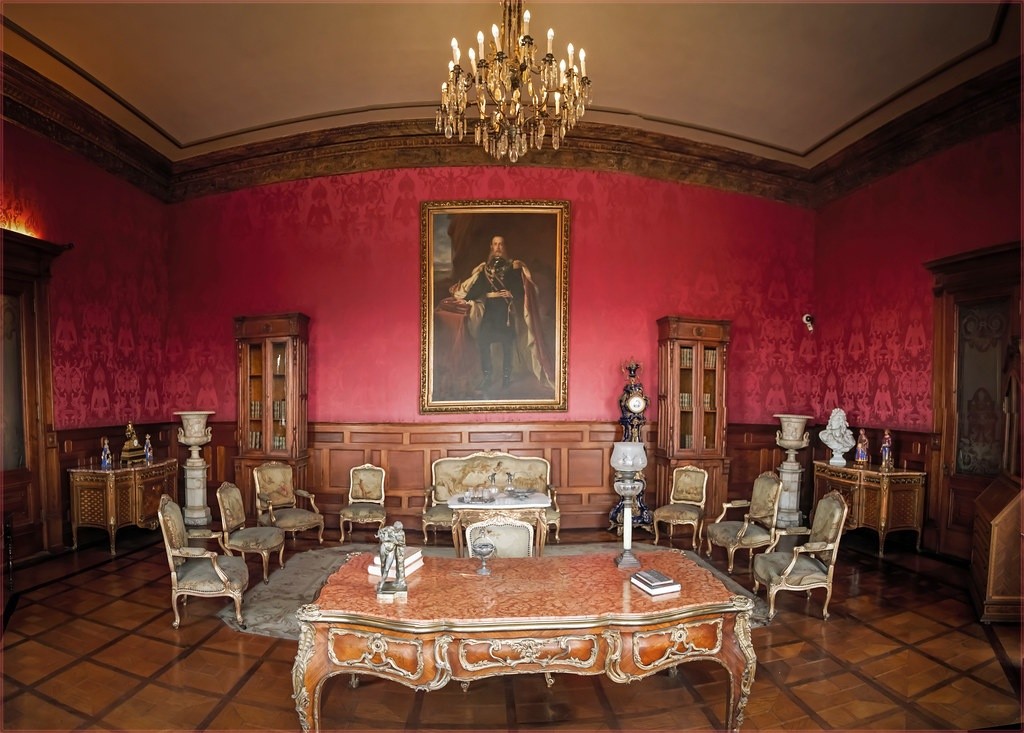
[432,1,595,166]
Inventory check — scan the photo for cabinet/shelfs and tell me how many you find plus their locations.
[658,316,727,461]
[234,314,307,459]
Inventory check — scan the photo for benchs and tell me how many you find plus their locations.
[422,451,562,548]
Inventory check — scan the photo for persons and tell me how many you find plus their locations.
[449,233,556,391]
[100,436,113,468]
[880,428,893,468]
[373,520,407,592]
[143,434,154,467]
[855,428,869,465]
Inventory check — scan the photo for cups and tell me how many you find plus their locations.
[463,486,490,503]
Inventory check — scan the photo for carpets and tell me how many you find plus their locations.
[215,537,778,641]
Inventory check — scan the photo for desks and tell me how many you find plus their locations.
[449,488,552,558]
[66,455,181,555]
[809,459,927,562]
[290,545,756,733]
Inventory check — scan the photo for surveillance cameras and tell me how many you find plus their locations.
[802,314,812,323]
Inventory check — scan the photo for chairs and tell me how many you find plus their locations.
[216,480,286,576]
[251,462,326,547]
[706,470,784,574]
[465,515,535,558]
[755,490,849,621]
[337,464,389,542]
[159,493,250,629]
[653,467,708,551]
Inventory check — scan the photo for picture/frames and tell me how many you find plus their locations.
[420,199,569,415]
